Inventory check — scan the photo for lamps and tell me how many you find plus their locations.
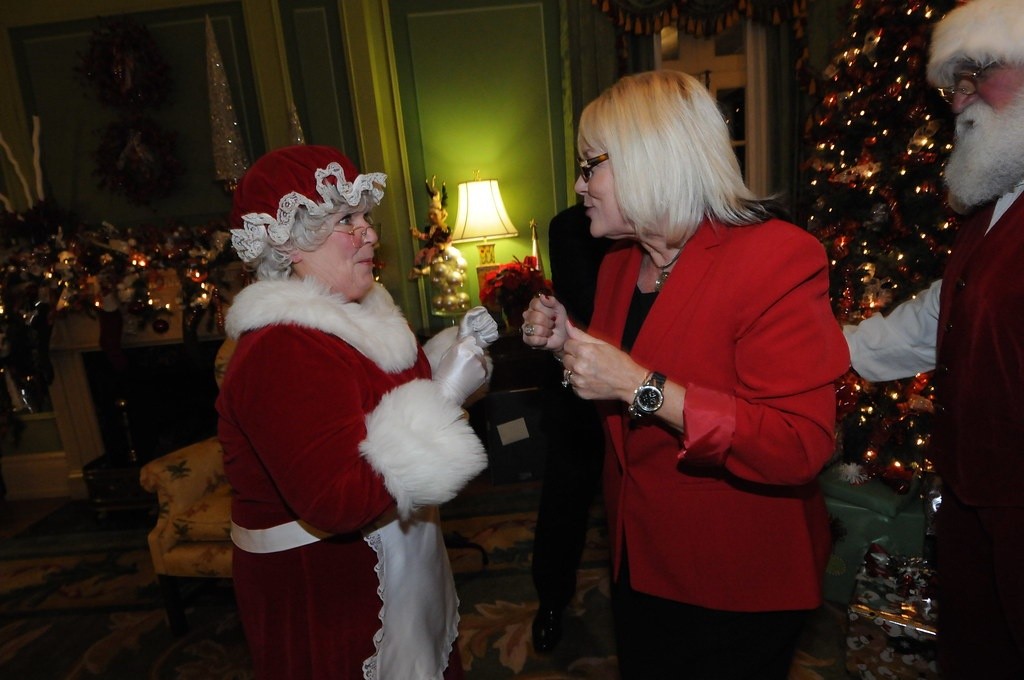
[447,177,520,327]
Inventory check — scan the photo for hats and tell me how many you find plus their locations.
[230,144,388,263]
[928,1,1024,87]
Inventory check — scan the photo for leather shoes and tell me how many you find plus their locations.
[532,605,563,656]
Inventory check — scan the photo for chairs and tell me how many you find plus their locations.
[140,436,235,636]
[819,449,930,607]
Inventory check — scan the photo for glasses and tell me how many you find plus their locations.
[575,154,609,184]
[940,58,996,106]
[330,222,383,249]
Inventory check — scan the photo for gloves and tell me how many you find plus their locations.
[457,306,499,349]
[437,336,489,407]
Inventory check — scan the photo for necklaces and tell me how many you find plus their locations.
[647,243,686,290]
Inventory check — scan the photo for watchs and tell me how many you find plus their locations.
[629,371,667,418]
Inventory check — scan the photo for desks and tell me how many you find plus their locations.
[417,324,558,482]
[843,556,941,680]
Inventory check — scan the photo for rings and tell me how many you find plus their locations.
[562,370,572,387]
[524,323,534,336]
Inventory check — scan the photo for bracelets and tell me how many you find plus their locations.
[553,353,564,362]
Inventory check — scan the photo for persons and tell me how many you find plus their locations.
[839,0,1024,680]
[520,67,850,680]
[214,144,501,680]
